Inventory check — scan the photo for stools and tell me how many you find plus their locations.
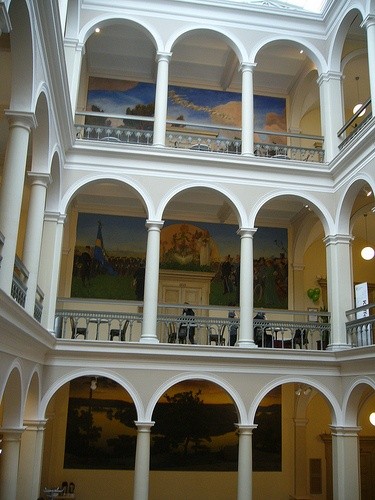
[168,336,175,343]
[216,338,225,345]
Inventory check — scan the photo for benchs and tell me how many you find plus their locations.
[165,126,219,151]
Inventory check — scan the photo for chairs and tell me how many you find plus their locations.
[69,316,87,340]
[166,321,186,344]
[110,320,129,341]
[61,481,75,493]
[209,323,227,346]
[292,328,306,348]
[253,313,274,347]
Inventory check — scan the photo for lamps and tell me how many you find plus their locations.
[363,185,372,196]
[353,75,366,117]
[295,384,312,396]
[360,213,375,261]
[90,377,99,390]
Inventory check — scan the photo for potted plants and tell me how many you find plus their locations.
[314,307,333,351]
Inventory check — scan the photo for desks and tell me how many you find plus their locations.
[88,319,109,340]
[37,492,76,500]
[182,323,208,344]
[100,136,122,143]
[271,154,292,160]
[268,328,288,348]
[189,144,211,152]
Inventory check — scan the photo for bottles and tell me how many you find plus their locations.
[69,482,74,493]
[62,481,67,495]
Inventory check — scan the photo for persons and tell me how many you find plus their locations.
[183,302,195,344]
[253,307,269,349]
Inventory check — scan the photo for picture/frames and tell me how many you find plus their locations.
[308,308,319,323]
